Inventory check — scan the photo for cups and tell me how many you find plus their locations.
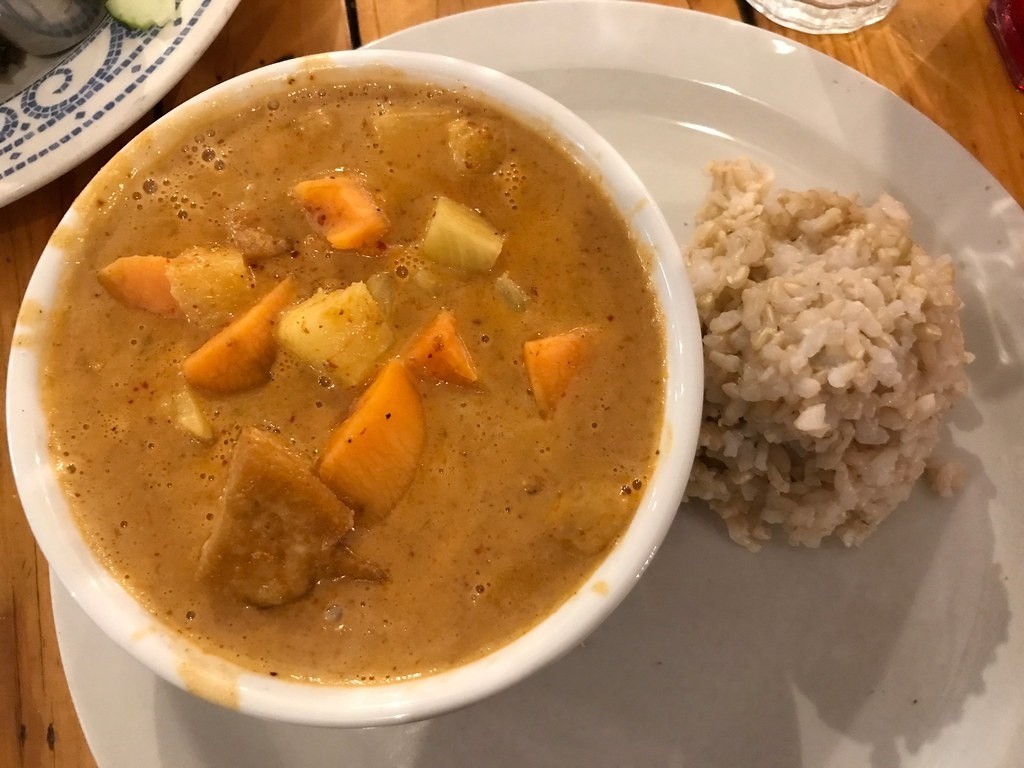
[747,0,898,36]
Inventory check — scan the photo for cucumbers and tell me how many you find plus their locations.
[106,0,179,32]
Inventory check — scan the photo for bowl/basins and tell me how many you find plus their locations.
[7,49,704,730]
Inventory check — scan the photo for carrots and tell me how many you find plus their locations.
[98,172,602,528]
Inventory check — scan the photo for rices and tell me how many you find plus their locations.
[676,154,978,547]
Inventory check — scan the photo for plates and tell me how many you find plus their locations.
[0,0,243,208]
[49,0,1024,767]
